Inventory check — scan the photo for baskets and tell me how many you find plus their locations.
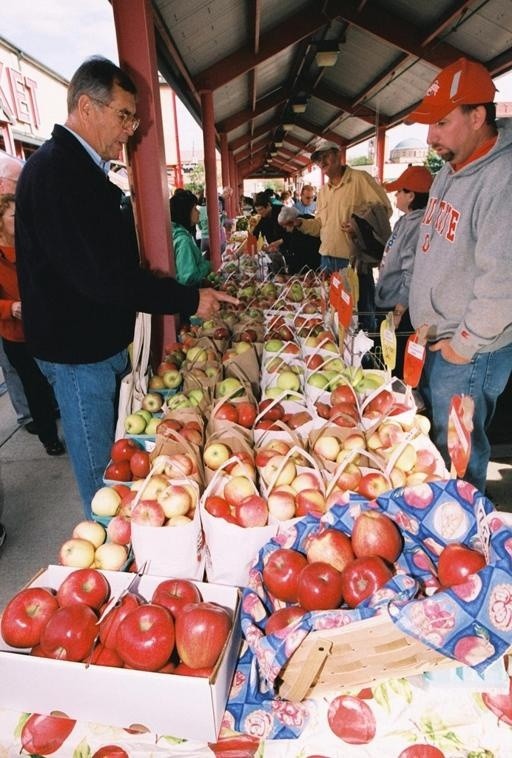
[277,613,512,704]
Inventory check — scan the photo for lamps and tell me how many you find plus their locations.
[261,35,348,169]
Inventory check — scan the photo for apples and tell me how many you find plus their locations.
[481,676,512,726]
[208,734,260,757]
[438,545,485,587]
[92,745,129,758]
[398,744,446,758]
[2,568,228,677]
[263,511,404,635]
[60,270,443,571]
[18,714,76,756]
[323,696,375,745]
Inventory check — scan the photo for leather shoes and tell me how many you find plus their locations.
[25,421,64,454]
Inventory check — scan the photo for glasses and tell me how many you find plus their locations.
[81,92,141,134]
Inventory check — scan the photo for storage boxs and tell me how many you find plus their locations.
[0,562,245,746]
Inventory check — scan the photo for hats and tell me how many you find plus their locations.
[402,58,496,126]
[311,141,341,160]
[383,166,434,193]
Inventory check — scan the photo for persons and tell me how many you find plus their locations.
[0,336,39,435]
[281,141,393,353]
[14,55,240,528]
[401,57,512,498]
[119,185,321,328]
[373,166,433,379]
[0,192,66,456]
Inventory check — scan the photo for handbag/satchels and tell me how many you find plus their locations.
[131,256,452,593]
[115,365,152,443]
